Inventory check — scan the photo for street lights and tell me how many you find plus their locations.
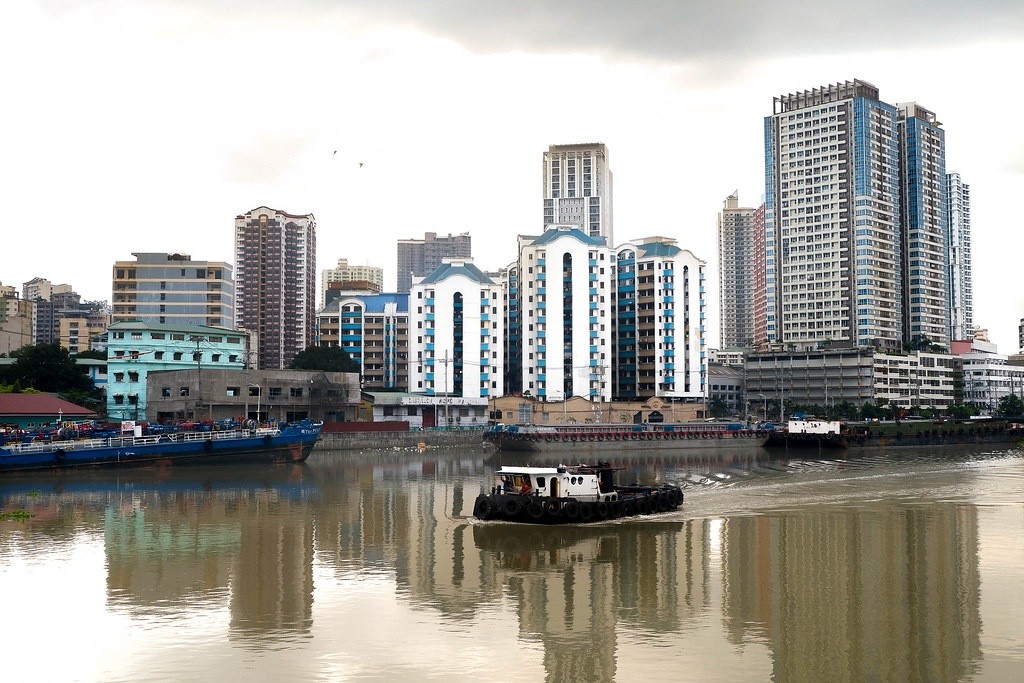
[759,394,767,423]
[557,390,567,423]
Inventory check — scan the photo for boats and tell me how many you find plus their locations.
[250,383,261,422]
[472,462,684,526]
[482,420,774,451]
[0,416,325,473]
[761,420,1024,451]
